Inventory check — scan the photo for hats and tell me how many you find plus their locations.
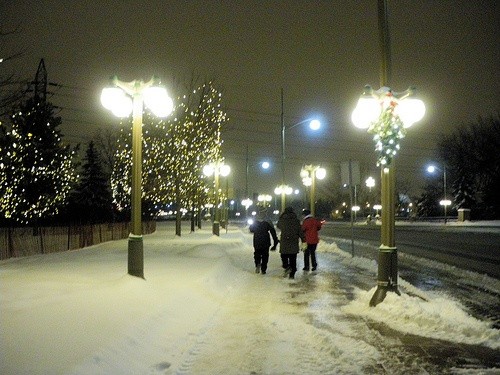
[257,212,265,221]
[303,209,311,215]
[284,207,295,213]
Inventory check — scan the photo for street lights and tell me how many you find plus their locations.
[364,176,376,222]
[299,162,327,219]
[274,183,294,215]
[99,73,173,281]
[352,0,427,309]
[279,87,321,213]
[426,162,448,224]
[258,193,273,214]
[203,161,231,236]
[240,198,254,219]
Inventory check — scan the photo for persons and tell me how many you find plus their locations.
[249,213,278,274]
[301,210,321,270]
[277,207,306,279]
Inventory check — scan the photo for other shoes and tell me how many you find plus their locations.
[289,275,296,279]
[312,267,317,271]
[303,267,309,270]
[262,271,266,274]
[256,264,261,274]
[283,268,291,278]
